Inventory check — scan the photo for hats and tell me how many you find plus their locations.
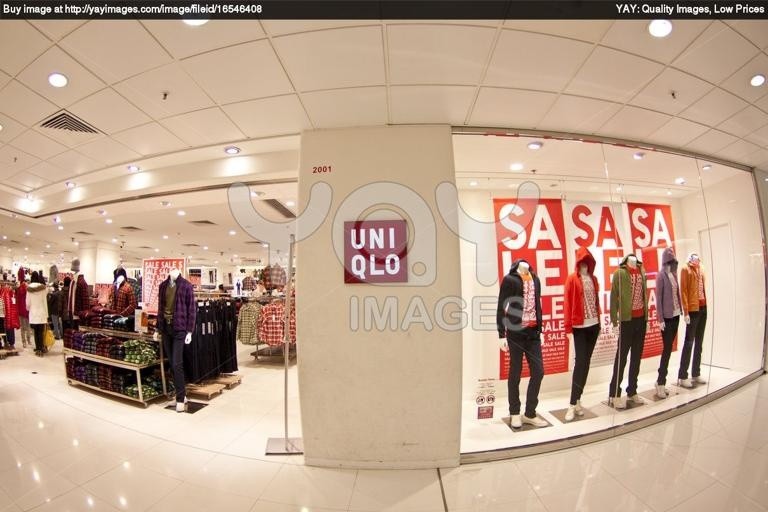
[50,265,58,277]
[71,258,79,271]
[18,267,25,281]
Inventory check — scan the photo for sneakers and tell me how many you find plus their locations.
[176,401,184,412]
[169,396,187,406]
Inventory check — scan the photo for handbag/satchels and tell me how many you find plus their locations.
[43,330,54,347]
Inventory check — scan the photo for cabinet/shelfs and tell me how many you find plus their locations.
[63,297,238,409]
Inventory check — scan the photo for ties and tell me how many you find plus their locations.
[70,280,75,320]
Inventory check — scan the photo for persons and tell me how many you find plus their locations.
[111,263,126,283]
[0,258,90,356]
[608,253,652,410]
[111,274,136,327]
[677,250,713,391]
[496,257,550,430]
[562,244,603,421]
[151,265,196,412]
[652,245,684,398]
